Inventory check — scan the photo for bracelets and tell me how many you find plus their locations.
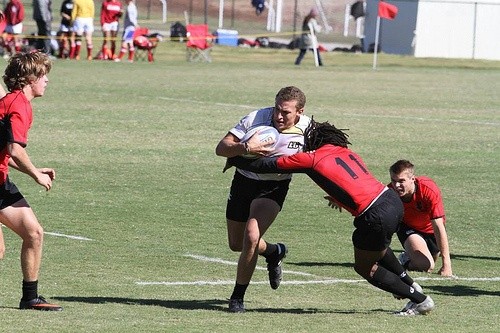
[244,142,250,155]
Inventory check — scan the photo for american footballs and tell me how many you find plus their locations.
[237,125,280,159]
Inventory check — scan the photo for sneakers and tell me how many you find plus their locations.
[229,299,244,312]
[265,243,288,290]
[20,295,62,311]
[400,282,434,316]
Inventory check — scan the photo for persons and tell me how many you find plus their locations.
[294,7,323,66]
[223,114,434,316]
[0,0,140,310]
[386,160,453,276]
[216,86,315,313]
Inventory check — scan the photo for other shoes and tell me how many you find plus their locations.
[399,253,420,272]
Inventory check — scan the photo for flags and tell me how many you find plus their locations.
[378,1,398,20]
